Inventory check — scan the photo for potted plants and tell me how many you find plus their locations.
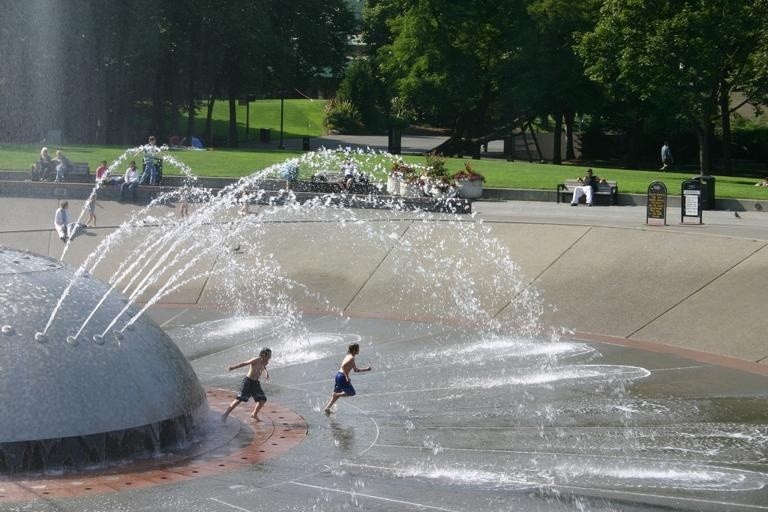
[387,152,486,197]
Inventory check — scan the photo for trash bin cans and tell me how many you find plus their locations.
[692,175,715,210]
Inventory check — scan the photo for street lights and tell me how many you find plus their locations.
[272,18,301,150]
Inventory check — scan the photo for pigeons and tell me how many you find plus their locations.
[233,245,240,252]
[734,211,741,218]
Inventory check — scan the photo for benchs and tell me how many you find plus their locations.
[312,172,367,194]
[557,179,617,206]
[31,161,89,182]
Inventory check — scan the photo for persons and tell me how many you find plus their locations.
[54,200,72,239]
[86,194,104,226]
[570,169,606,207]
[659,142,674,173]
[325,343,371,413]
[33,135,204,203]
[336,160,357,195]
[221,347,271,424]
